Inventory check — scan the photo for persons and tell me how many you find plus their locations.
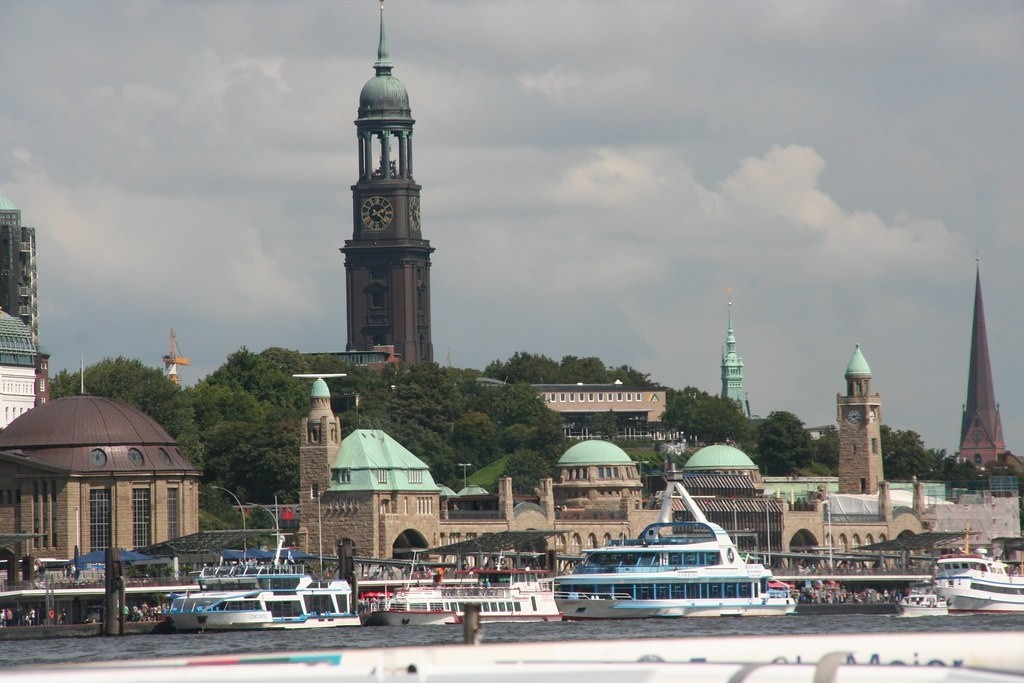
[0,547,1022,627]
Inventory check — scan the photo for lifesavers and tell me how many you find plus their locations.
[981,572,985,577]
[1009,577,1012,583]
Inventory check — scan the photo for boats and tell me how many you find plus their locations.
[895,583,950,617]
[357,564,566,626]
[0,571,210,641]
[933,551,1023,615]
[552,465,799,619]
[161,536,363,632]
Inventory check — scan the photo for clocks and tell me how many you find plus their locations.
[361,196,393,230]
[409,197,421,230]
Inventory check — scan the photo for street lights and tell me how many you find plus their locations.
[246,501,280,564]
[822,500,833,570]
[458,463,472,488]
[210,485,248,565]
